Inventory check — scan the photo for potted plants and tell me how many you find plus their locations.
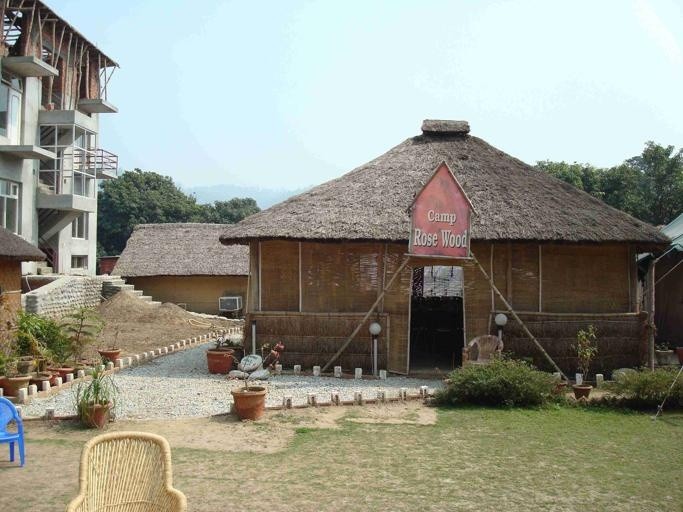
[551,378,568,397]
[655,342,674,365]
[0,299,121,428]
[572,324,599,400]
[207,316,243,374]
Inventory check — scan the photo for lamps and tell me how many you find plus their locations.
[369,322,381,376]
[494,313,507,340]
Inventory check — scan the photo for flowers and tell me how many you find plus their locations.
[222,341,286,390]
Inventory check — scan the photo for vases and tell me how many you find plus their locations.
[230,387,266,422]
[675,347,683,365]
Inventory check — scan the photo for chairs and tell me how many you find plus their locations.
[0,397,25,466]
[65,430,187,512]
[461,335,505,368]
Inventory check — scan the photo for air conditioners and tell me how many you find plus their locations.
[218,296,243,311]
